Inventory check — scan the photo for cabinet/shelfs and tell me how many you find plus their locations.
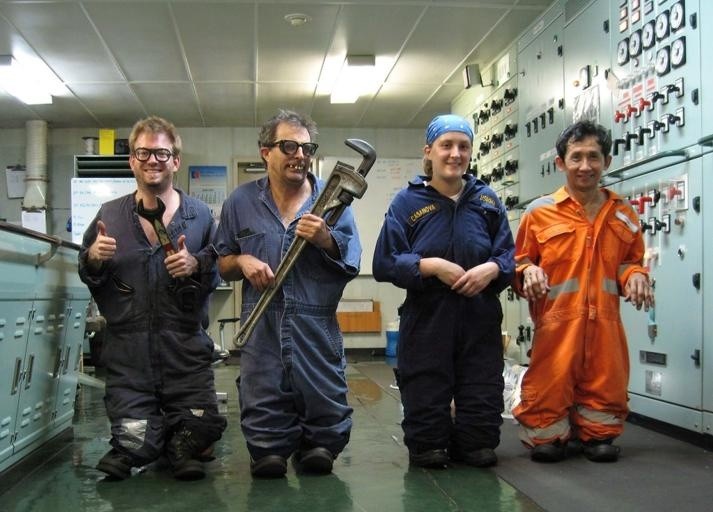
[0,228,95,472]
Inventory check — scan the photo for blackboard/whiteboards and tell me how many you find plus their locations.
[319,156,424,276]
[71,177,139,248]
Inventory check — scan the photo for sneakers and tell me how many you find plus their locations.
[531,441,567,461]
[251,453,287,479]
[96,446,133,478]
[292,447,334,476]
[465,450,498,467]
[584,439,620,462]
[160,433,205,480]
[408,449,451,469]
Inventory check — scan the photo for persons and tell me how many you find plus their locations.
[206,110,360,476]
[371,115,516,469]
[510,121,655,462]
[77,117,226,479]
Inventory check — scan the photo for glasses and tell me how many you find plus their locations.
[133,147,174,162]
[263,139,318,158]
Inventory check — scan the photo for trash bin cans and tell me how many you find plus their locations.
[386,330,399,358]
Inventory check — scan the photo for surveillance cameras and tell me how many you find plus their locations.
[463,63,481,89]
